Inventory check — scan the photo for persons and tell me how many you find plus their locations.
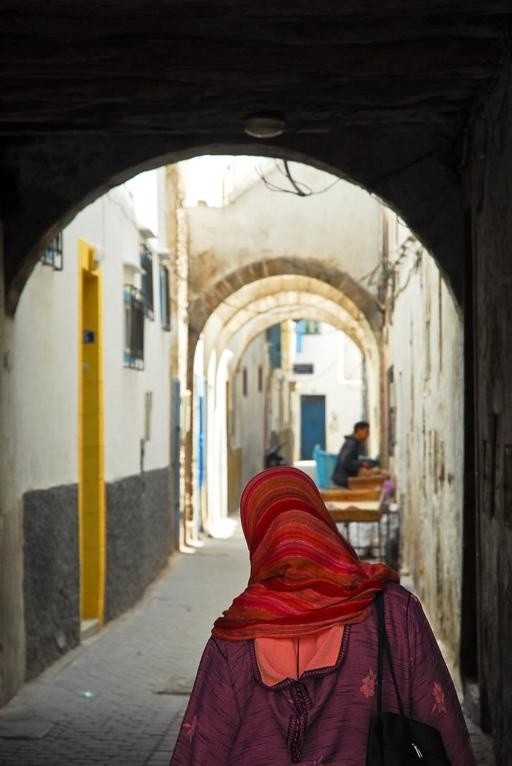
[169,466,475,765]
[331,422,369,487]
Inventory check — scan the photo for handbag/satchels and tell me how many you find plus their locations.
[363,712,453,766]
